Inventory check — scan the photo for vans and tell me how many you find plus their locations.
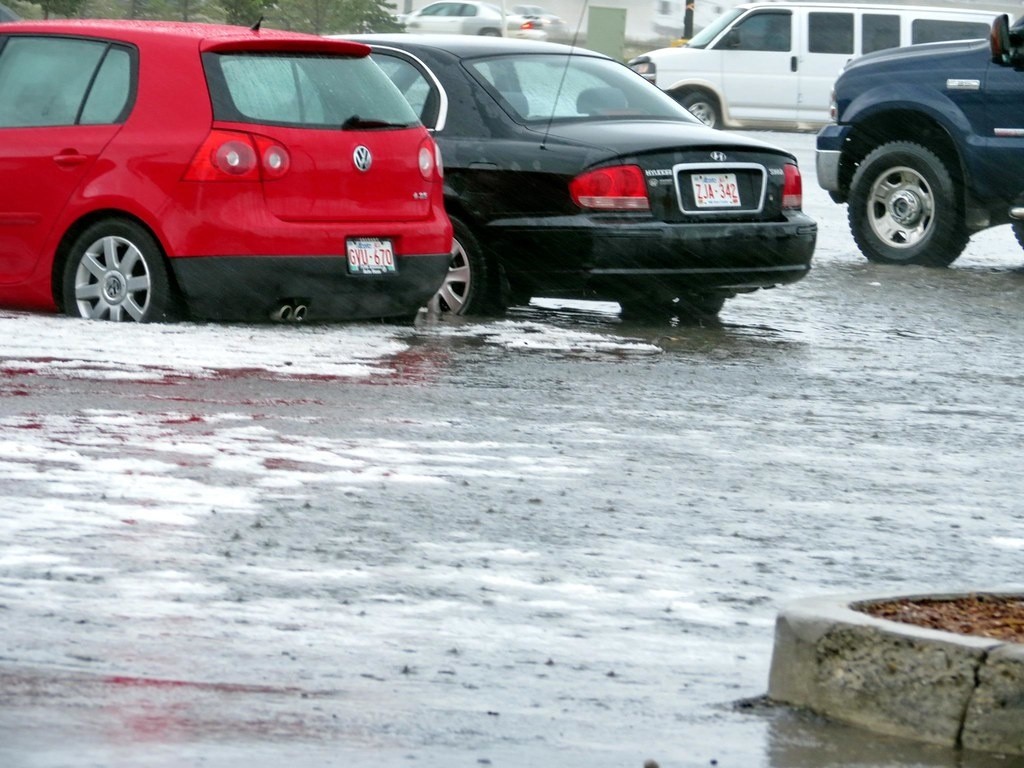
[620,4,1019,131]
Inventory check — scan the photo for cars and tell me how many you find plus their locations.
[0,17,455,329]
[308,32,818,320]
[407,1,568,45]
[818,9,1024,268]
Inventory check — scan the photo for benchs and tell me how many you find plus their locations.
[500,87,627,119]
